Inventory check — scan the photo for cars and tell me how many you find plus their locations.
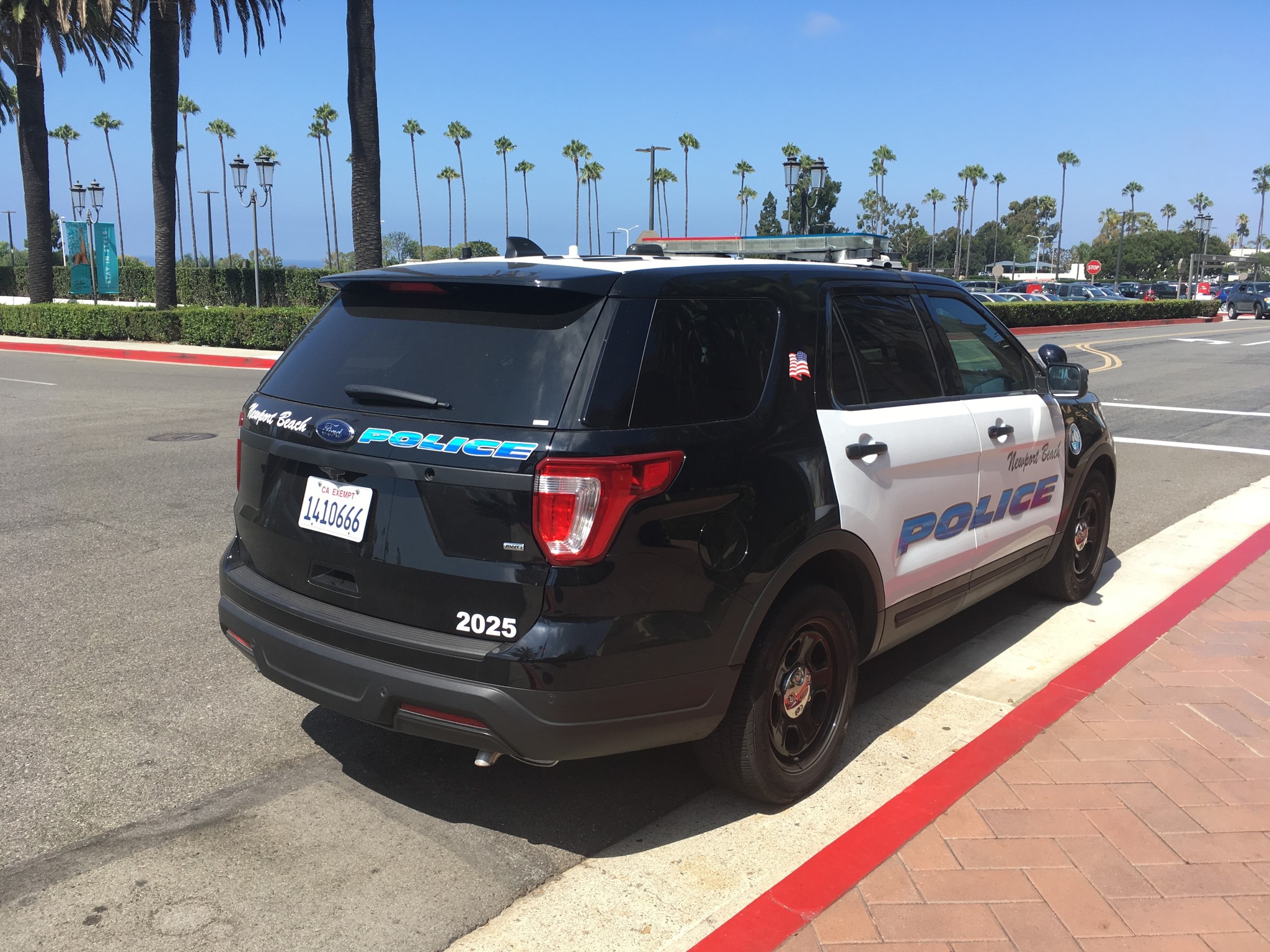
[951,277,1270,320]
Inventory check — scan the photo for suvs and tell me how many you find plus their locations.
[208,224,1120,815]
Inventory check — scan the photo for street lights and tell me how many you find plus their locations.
[635,144,671,230]
[923,236,938,268]
[69,177,104,306]
[1194,211,1214,300]
[782,151,830,235]
[606,231,621,255]
[197,190,220,268]
[1112,211,1134,293]
[1026,235,1054,279]
[228,150,276,311]
[1200,226,1218,283]
[1,210,17,296]
[57,216,66,267]
[617,225,639,251]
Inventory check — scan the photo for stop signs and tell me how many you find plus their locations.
[1087,260,1102,274]
